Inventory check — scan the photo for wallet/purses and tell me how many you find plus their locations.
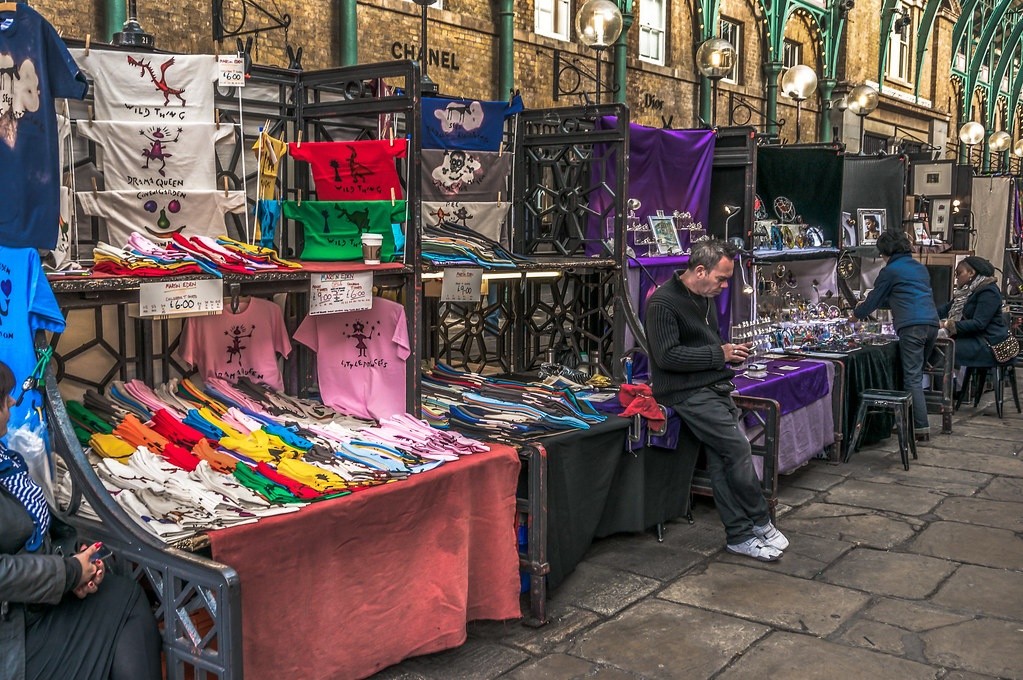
[708,380,736,397]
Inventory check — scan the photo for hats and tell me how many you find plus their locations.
[876,232,911,256]
[964,256,995,277]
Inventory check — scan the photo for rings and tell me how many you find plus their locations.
[733,349,738,354]
[731,343,736,348]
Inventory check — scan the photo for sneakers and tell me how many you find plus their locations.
[726,537,783,562]
[752,519,790,550]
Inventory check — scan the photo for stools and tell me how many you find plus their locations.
[844,389,918,470]
[954,359,1021,419]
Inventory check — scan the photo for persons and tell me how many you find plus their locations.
[937,256,1012,408]
[848,228,941,441]
[0,359,164,680]
[864,215,880,239]
[643,238,790,562]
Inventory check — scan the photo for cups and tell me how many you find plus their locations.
[361,233,383,265]
[544,348,601,377]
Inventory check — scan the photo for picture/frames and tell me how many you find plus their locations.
[928,197,951,233]
[857,207,888,246]
[647,215,683,254]
[909,158,955,198]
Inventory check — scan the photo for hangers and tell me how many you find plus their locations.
[0,0,17,12]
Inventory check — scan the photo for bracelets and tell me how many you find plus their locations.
[943,319,949,328]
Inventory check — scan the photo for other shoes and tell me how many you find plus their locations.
[893,427,929,442]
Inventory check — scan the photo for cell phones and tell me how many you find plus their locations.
[71,544,113,564]
[747,346,756,351]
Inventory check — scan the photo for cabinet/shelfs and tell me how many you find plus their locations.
[40,44,1021,679]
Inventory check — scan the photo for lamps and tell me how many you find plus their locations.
[1014,138,1023,175]
[845,84,879,154]
[953,199,976,233]
[960,122,985,163]
[696,37,736,125]
[577,0,622,103]
[737,254,754,294]
[837,259,856,280]
[627,253,660,290]
[989,131,1011,173]
[782,64,818,142]
[722,204,741,243]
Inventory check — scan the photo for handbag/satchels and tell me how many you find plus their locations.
[991,336,1020,362]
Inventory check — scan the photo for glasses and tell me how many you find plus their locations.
[879,253,884,259]
